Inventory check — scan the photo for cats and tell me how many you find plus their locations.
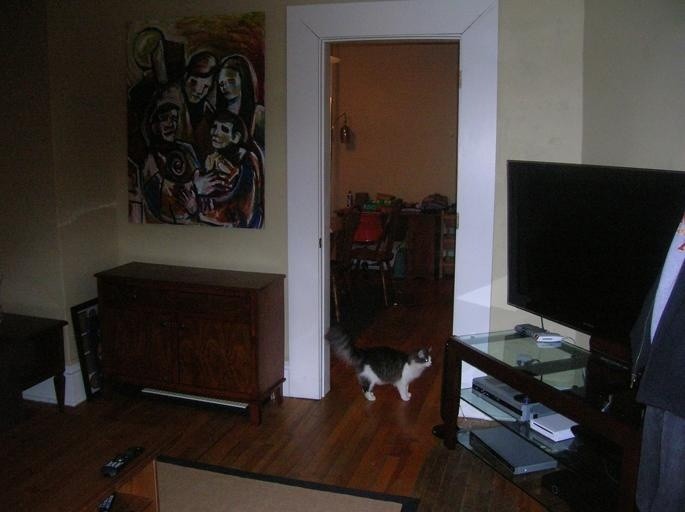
[324,324,432,402]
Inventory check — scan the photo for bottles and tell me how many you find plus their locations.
[346,190,353,208]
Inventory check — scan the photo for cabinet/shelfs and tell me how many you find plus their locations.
[395,210,456,282]
[89,261,286,428]
[437,328,646,512]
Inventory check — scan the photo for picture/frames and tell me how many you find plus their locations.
[69,297,104,401]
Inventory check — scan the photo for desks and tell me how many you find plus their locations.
[0,312,68,438]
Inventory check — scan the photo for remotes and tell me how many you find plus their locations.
[101,445,144,477]
[97,492,115,511]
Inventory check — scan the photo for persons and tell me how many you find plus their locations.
[628,212,685,511]
[126,26,266,228]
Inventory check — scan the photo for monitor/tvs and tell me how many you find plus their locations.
[506,158,685,347]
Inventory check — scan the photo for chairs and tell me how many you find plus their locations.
[331,199,403,325]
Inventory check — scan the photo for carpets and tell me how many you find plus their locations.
[153,451,421,512]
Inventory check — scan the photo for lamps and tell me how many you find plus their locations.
[330,110,351,143]
